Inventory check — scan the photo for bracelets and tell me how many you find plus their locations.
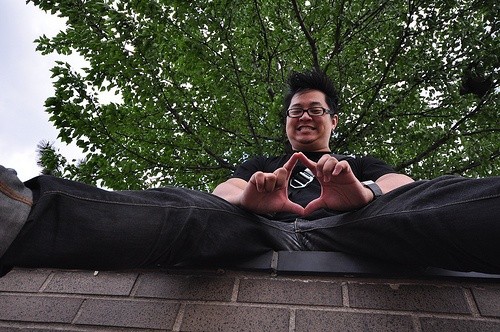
[361,179,381,200]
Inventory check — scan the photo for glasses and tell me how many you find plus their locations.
[285,106,334,117]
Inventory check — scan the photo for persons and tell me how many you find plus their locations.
[0,70,500,274]
